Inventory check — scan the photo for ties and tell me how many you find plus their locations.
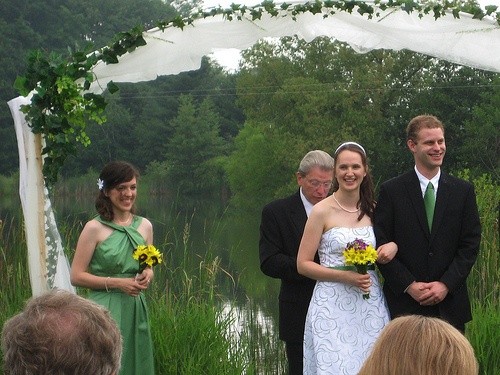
[424,182,436,235]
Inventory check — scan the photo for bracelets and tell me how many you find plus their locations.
[104,276,112,293]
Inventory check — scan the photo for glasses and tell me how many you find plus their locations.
[310,181,333,189]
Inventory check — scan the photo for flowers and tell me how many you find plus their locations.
[97,178,104,190]
[133,244,163,274]
[341,239,379,300]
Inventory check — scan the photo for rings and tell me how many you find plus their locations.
[147,279,150,283]
[380,258,386,262]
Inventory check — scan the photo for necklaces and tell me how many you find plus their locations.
[332,192,364,214]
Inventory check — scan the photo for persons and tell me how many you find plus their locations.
[358,315,481,375]
[296,142,400,374]
[371,113,482,337]
[0,287,123,375]
[258,149,335,375]
[69,159,157,375]
